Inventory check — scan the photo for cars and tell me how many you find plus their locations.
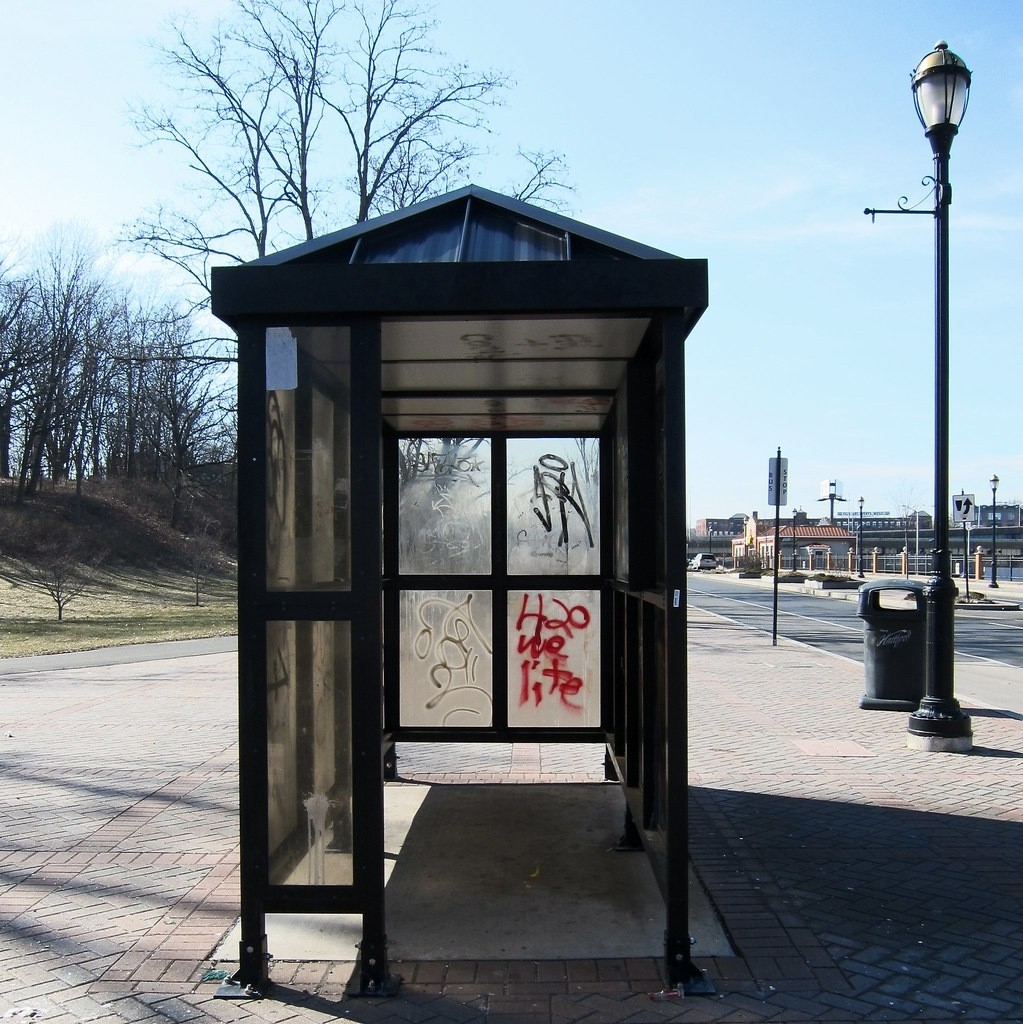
[693,553,716,569]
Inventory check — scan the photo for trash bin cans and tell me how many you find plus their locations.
[856,580,928,712]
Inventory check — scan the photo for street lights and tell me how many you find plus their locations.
[858,496,865,578]
[744,516,748,557]
[905,41,977,751]
[988,474,999,588]
[709,526,713,553]
[792,508,797,571]
